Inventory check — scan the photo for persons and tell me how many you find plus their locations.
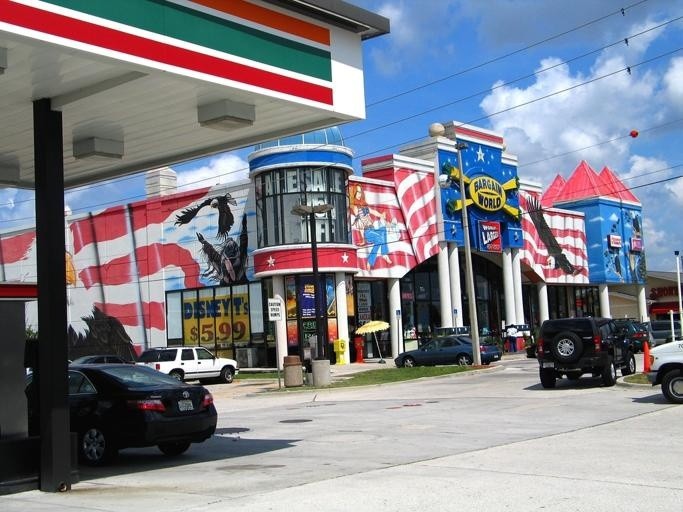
[504,324,518,353]
[347,181,393,272]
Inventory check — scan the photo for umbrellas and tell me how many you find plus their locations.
[354,318,392,365]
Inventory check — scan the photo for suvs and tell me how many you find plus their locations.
[537,318,636,388]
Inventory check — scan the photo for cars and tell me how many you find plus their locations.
[611,318,683,404]
[394,336,501,367]
[25,346,238,467]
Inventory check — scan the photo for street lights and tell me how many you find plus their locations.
[290,204,333,360]
[455,142,481,365]
[675,251,683,340]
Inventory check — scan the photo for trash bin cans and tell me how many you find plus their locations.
[312,359,331,387]
[283,356,303,387]
